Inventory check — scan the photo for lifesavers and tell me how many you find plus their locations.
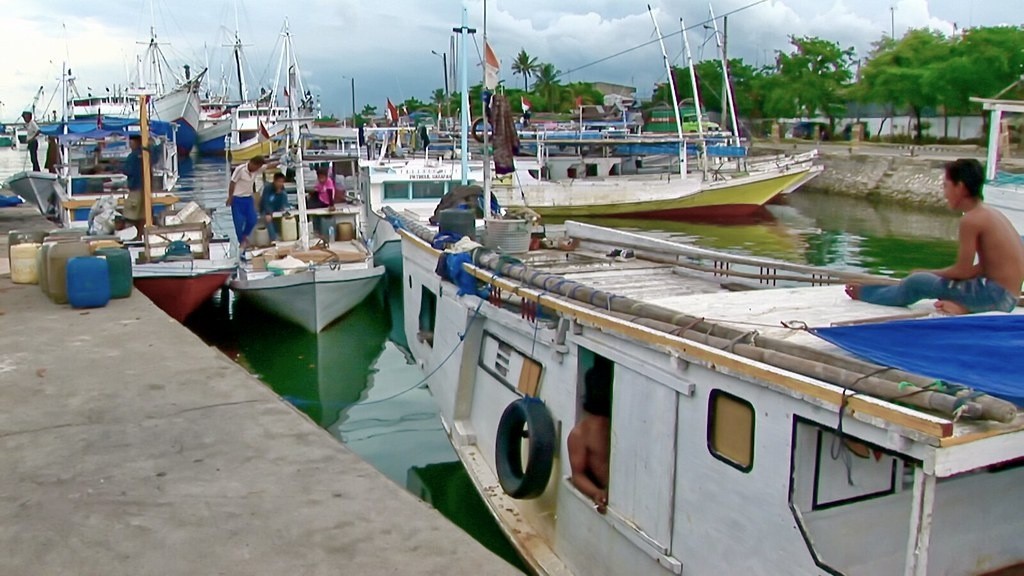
[494,392,554,499]
[471,115,491,143]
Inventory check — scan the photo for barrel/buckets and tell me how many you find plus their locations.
[440,203,477,242]
[8,231,49,271]
[10,243,42,284]
[281,211,298,242]
[152,203,166,226]
[93,247,132,298]
[89,241,124,254]
[483,217,532,254]
[81,235,121,244]
[530,224,546,250]
[65,255,110,308]
[253,223,270,247]
[38,226,86,292]
[46,243,91,305]
[319,215,336,242]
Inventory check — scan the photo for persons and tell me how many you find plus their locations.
[260,172,290,245]
[23,112,40,172]
[844,158,1024,316]
[226,156,265,249]
[567,364,614,515]
[295,167,335,234]
[44,136,59,175]
[123,133,145,242]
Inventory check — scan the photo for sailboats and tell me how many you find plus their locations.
[1,1,825,229]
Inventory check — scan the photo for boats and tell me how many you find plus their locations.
[968,75,1024,237]
[77,91,237,326]
[208,12,388,337]
[376,0,1024,574]
[355,5,477,283]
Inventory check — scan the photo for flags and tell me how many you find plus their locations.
[482,37,499,90]
[520,95,533,114]
[258,119,270,144]
[386,98,400,122]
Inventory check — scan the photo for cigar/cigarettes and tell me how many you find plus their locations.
[593,505,599,509]
